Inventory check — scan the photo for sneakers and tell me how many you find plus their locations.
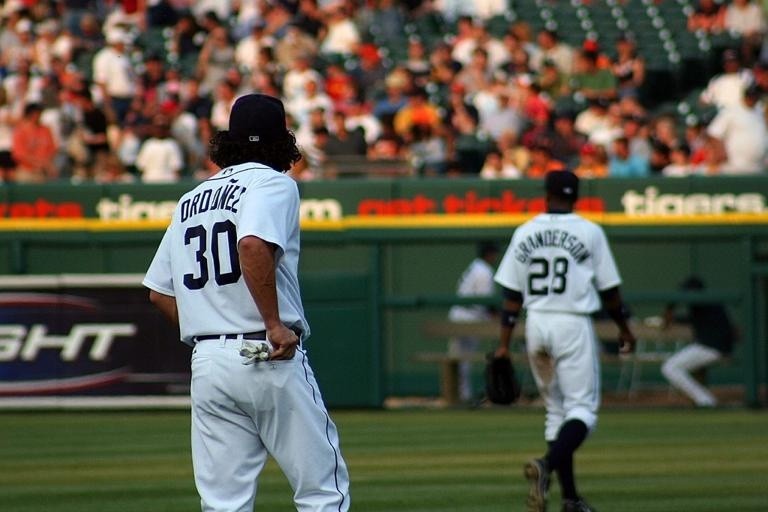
[524,458,552,512]
[562,497,596,512]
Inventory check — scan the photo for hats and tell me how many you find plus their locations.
[228,94,286,142]
[545,169,578,202]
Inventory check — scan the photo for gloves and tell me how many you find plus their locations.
[241,342,270,365]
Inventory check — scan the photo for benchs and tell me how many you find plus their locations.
[420,324,693,406]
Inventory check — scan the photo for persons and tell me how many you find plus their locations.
[493,172,637,512]
[142,94,350,512]
[448,238,499,408]
[660,275,733,408]
[0,0,767,185]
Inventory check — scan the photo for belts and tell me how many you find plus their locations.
[191,327,265,341]
